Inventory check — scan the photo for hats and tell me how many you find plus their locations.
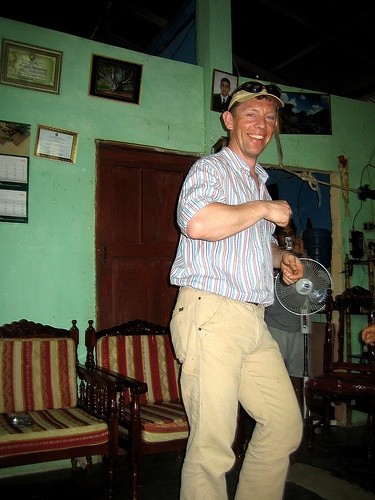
[227,80,284,112]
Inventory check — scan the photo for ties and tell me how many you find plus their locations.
[223,99,225,104]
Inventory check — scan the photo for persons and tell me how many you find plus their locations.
[170,80,302,500]
[362,324,375,345]
[264,221,320,419]
[212,78,231,112]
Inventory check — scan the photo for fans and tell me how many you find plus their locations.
[275,258,333,420]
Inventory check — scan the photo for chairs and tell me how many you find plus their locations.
[304,287,375,460]
[0,320,189,500]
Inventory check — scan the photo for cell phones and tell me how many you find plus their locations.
[284,237,293,251]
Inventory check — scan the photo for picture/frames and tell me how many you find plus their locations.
[88,53,144,105]
[211,69,238,113]
[277,91,332,136]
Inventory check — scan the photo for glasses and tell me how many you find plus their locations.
[228,81,283,99]
